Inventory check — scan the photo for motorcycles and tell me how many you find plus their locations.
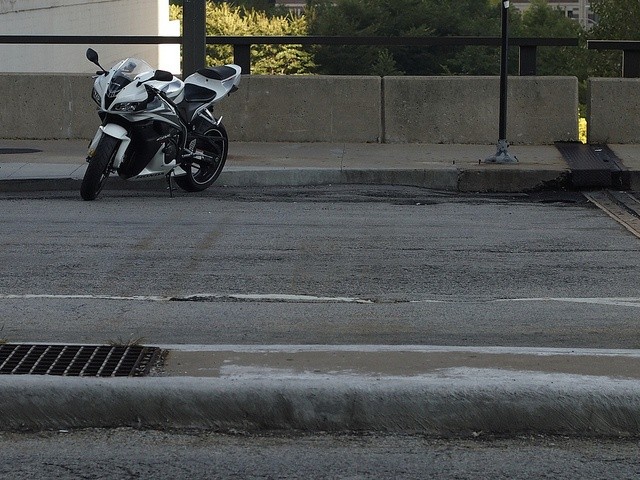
[81,47,242,201]
